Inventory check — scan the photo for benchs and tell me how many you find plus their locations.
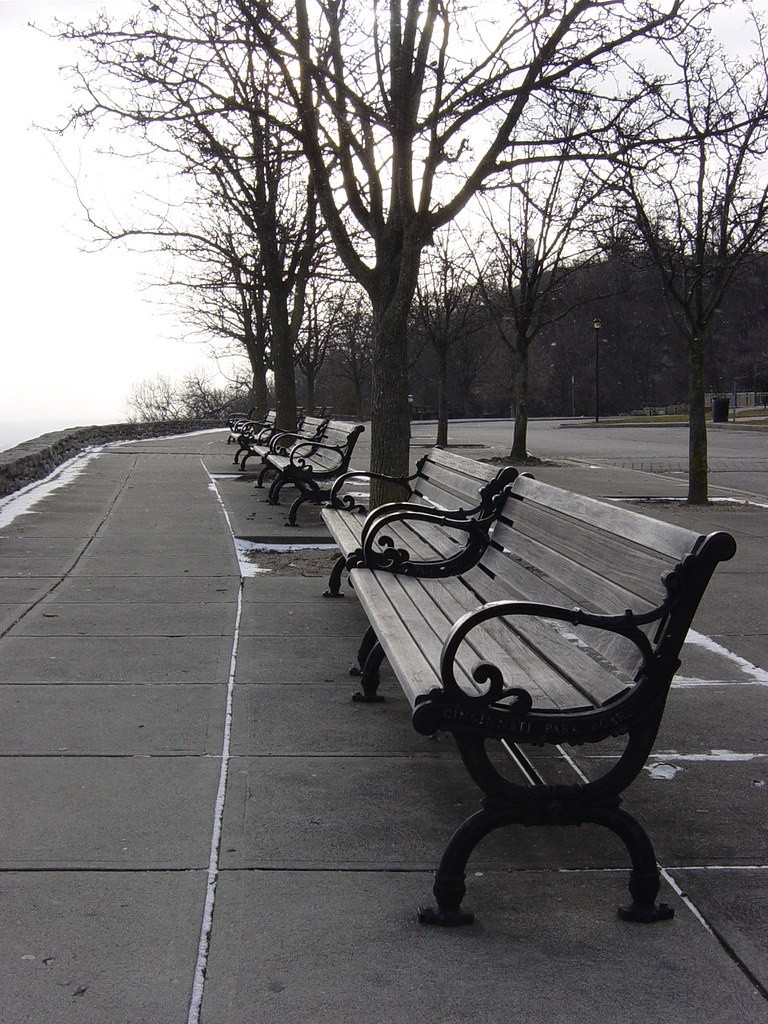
[323,445,518,679]
[348,469,736,923]
[227,406,365,526]
